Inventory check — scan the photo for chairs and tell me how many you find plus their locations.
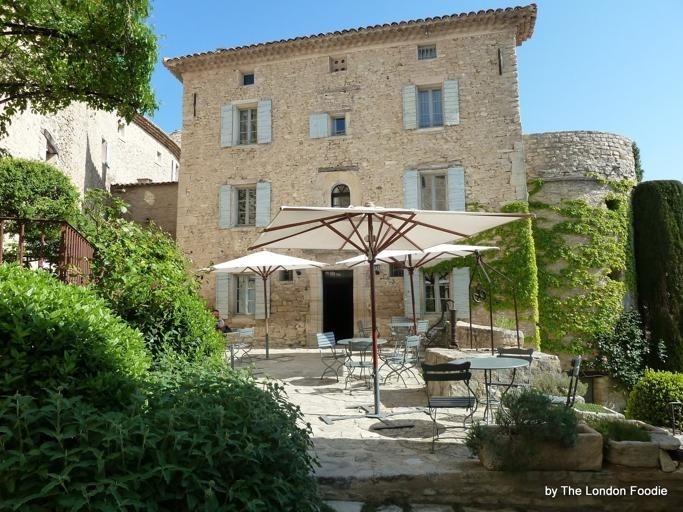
[223,330,256,363]
[419,345,586,455]
[315,316,431,395]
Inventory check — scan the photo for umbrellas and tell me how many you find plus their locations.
[333,245,500,354]
[246,204,537,416]
[195,251,330,360]
